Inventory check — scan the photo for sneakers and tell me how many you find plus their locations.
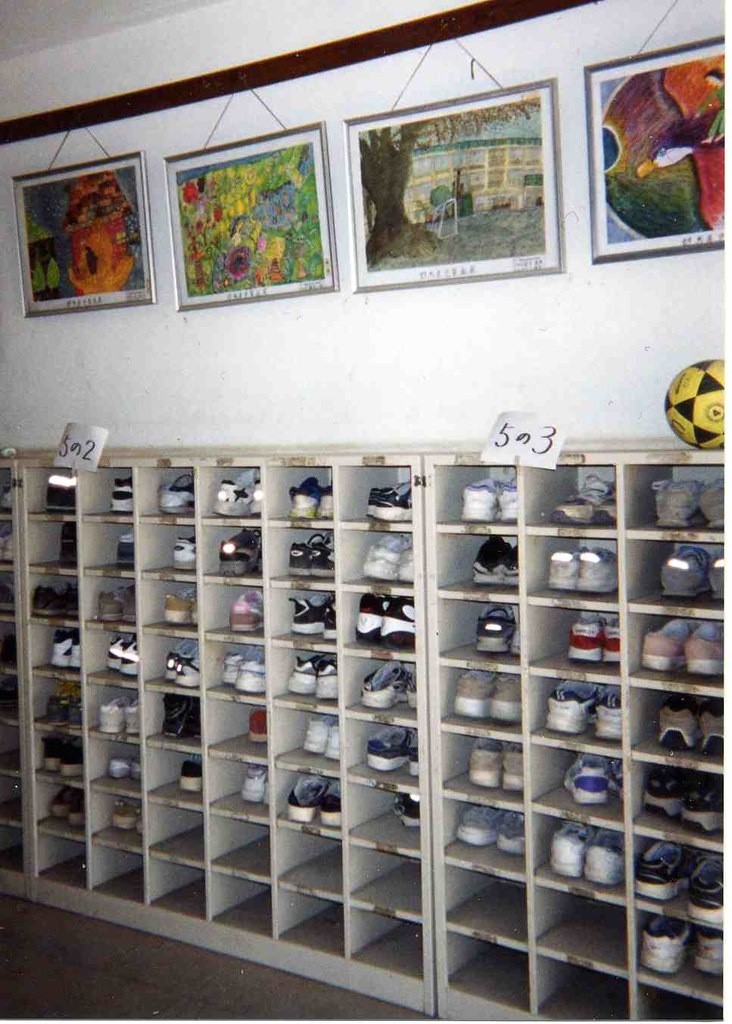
[0,677,18,709]
[98,696,139,734]
[660,546,724,599]
[474,602,521,656]
[179,760,202,792]
[31,581,79,616]
[111,799,142,835]
[452,671,521,723]
[220,650,265,694]
[106,635,137,676]
[641,618,724,675]
[172,536,196,570]
[0,483,13,509]
[564,754,625,806]
[247,709,267,744]
[546,546,618,592]
[107,755,141,781]
[46,694,82,729]
[391,793,420,827]
[40,735,83,777]
[49,788,85,827]
[211,477,262,517]
[0,537,13,562]
[354,593,416,647]
[302,715,340,762]
[286,477,333,519]
[471,535,518,585]
[108,477,133,513]
[116,533,134,569]
[656,696,723,755]
[567,611,621,662]
[643,767,723,833]
[285,774,341,827]
[49,628,81,670]
[362,534,415,583]
[468,738,524,793]
[633,841,724,925]
[650,476,725,527]
[550,475,616,524]
[548,820,624,886]
[157,474,194,514]
[456,806,526,855]
[285,652,338,700]
[365,726,418,776]
[638,914,722,975]
[1,635,17,663]
[288,591,337,640]
[360,661,417,709]
[161,694,201,737]
[97,584,136,622]
[218,528,261,576]
[162,587,198,625]
[458,479,517,522]
[45,485,75,512]
[288,531,335,578]
[545,680,623,741]
[164,639,200,688]
[0,585,14,612]
[239,765,268,806]
[229,590,263,632]
[366,481,412,521]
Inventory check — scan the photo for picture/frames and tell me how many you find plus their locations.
[11,149,158,317]
[584,33,725,264]
[343,78,568,294]
[164,120,340,312]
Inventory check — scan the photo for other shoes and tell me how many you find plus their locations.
[58,523,76,568]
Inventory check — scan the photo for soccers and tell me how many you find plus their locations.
[663,359,724,450]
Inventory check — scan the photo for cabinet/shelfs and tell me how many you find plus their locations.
[0,449,724,1021]
